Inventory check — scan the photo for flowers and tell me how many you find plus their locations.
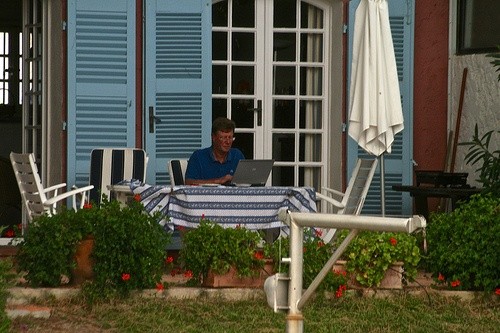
[416,192,500,296]
[6,193,174,292]
[263,228,349,297]
[179,213,270,278]
[332,228,421,287]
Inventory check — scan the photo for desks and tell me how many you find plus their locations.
[106,180,317,243]
[393,185,500,225]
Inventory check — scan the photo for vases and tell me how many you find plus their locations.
[203,259,275,289]
[333,261,403,290]
[69,233,101,284]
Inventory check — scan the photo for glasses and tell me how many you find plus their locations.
[216,135,235,142]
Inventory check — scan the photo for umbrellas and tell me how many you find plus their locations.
[347,0,404,217]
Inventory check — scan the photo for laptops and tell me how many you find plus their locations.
[225,160,275,186]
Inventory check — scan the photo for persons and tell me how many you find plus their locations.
[184,117,245,185]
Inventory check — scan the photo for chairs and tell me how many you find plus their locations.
[315,159,377,244]
[87,148,149,204]
[10,151,94,224]
[168,158,191,187]
